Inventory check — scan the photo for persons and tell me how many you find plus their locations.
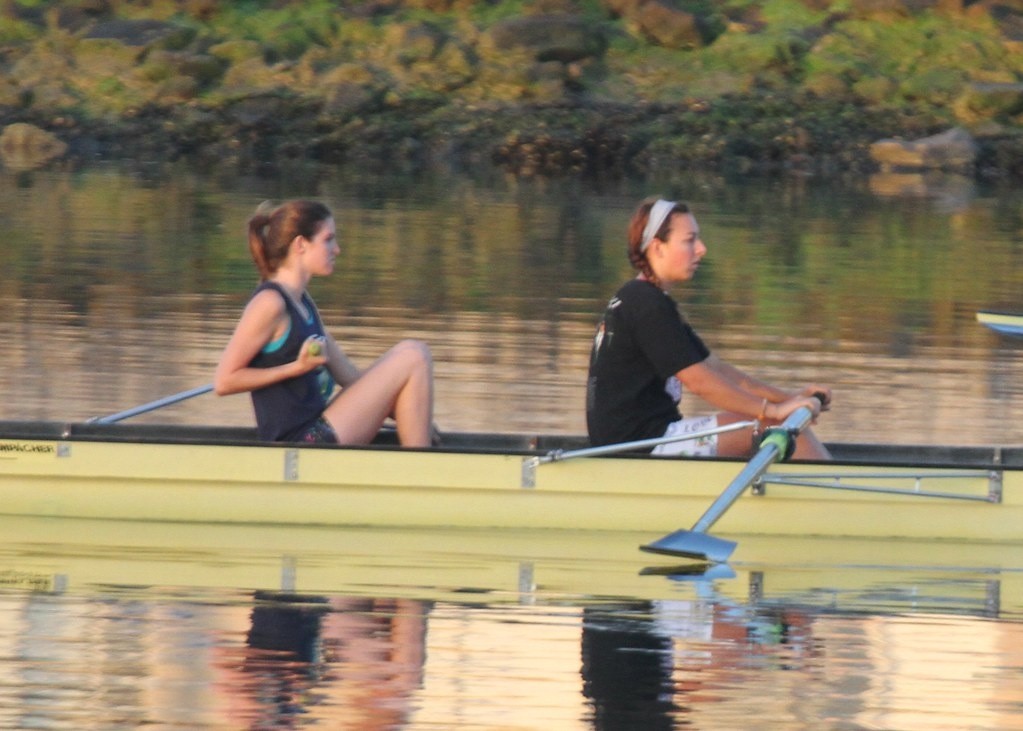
[213,198,432,447]
[585,197,837,460]
[205,593,813,731]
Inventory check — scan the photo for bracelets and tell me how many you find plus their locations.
[758,398,767,421]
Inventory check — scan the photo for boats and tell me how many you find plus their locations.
[0,418,1023,550]
[978,311,1023,341]
[0,537,1023,628]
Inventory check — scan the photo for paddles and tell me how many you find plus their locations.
[637,390,828,565]
[308,341,324,358]
